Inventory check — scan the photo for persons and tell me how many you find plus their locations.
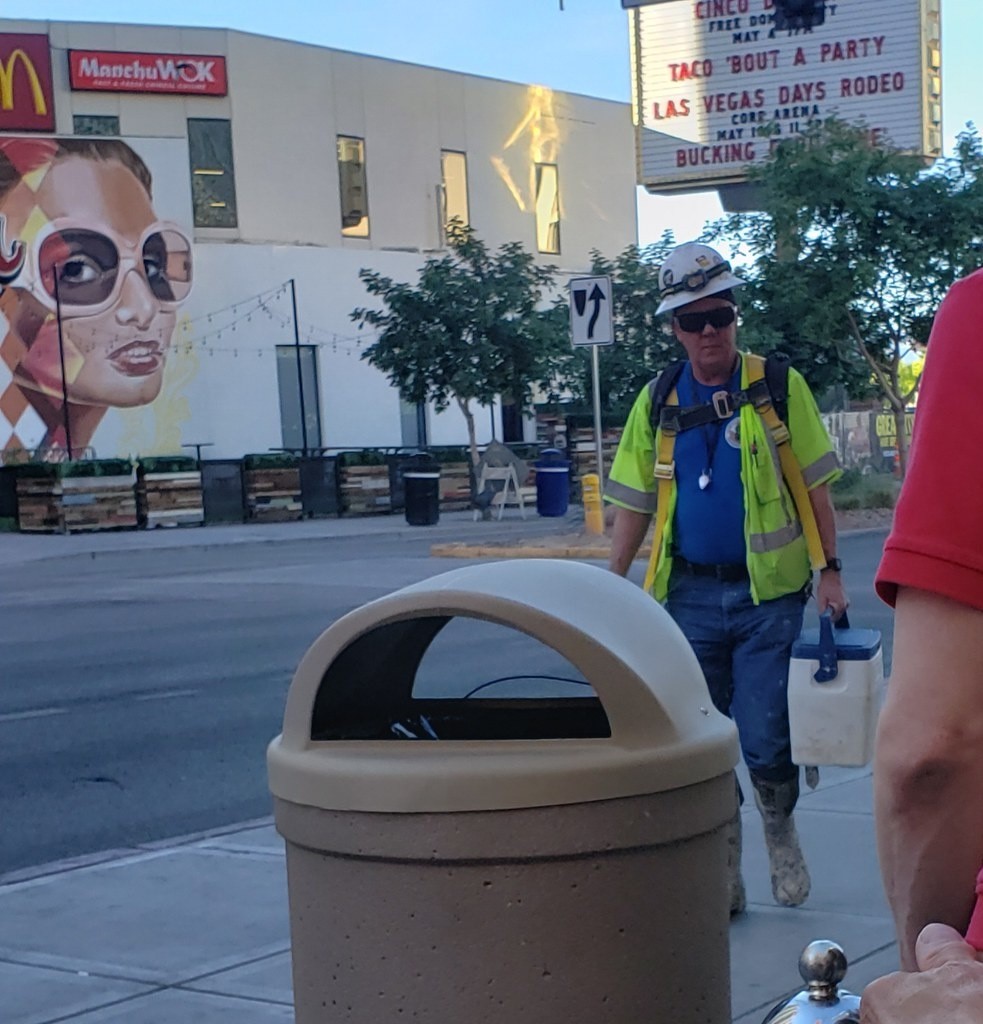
[602,242,851,919]
[868,266,983,969]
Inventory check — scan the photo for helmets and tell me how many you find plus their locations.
[655,242,746,315]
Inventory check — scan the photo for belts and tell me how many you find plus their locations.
[673,555,749,582]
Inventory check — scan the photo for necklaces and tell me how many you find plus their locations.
[691,351,738,490]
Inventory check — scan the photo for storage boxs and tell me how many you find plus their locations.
[788,605,882,770]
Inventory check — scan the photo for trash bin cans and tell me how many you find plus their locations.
[534,448,572,517]
[401,453,440,526]
[265,558,741,1023]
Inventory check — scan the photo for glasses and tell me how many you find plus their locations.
[670,306,740,333]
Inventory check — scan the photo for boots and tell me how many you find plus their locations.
[753,786,811,907]
[721,793,747,917]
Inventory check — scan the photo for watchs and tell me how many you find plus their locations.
[820,558,843,571]
